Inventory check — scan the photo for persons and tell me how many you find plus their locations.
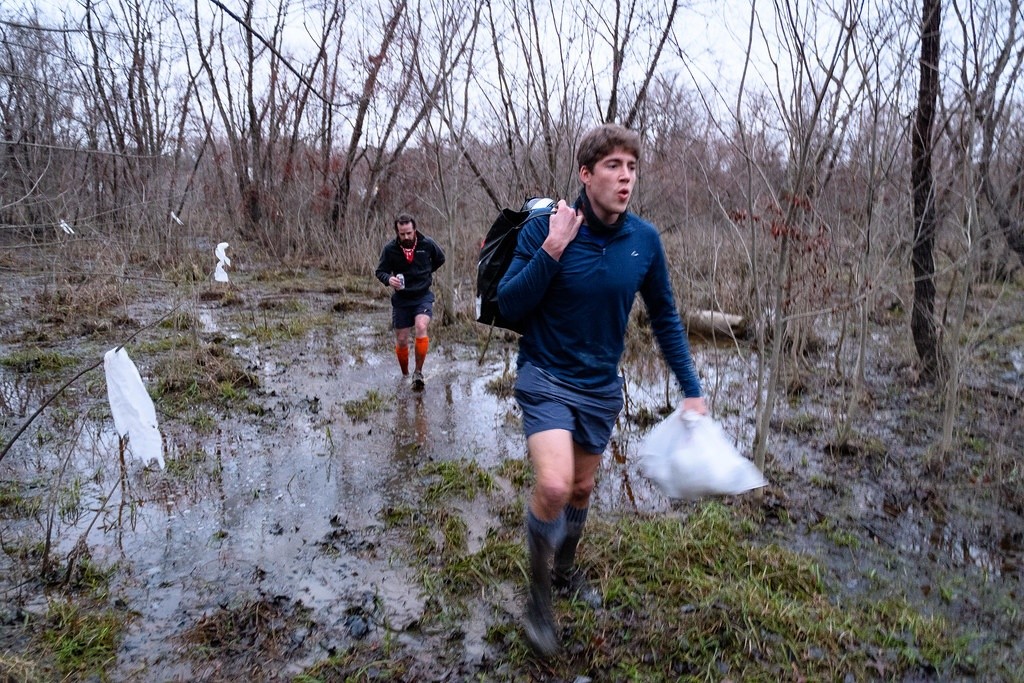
[375,214,446,385]
[497,124,709,657]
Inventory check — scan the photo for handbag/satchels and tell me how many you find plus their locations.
[474,207,557,335]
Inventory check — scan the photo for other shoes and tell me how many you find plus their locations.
[412,370,425,386]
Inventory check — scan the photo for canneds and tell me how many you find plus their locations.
[396,274,405,289]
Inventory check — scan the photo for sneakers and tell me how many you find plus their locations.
[550,563,602,608]
[523,602,556,657]
